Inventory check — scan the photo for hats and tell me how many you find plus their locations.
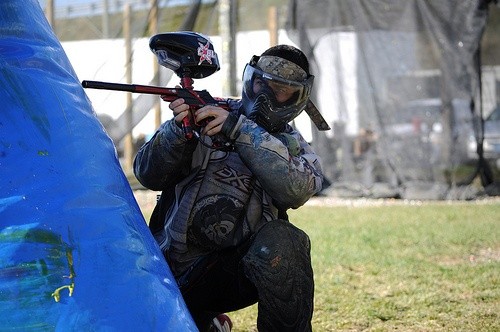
[256,44,309,83]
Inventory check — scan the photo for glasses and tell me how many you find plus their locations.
[243,63,304,111]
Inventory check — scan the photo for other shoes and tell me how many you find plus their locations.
[208,314,233,332]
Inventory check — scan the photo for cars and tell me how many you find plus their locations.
[376,94,500,162]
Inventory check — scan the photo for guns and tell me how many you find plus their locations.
[81,30,236,141]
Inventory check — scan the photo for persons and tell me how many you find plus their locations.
[132,45,333,331]
[351,130,379,166]
